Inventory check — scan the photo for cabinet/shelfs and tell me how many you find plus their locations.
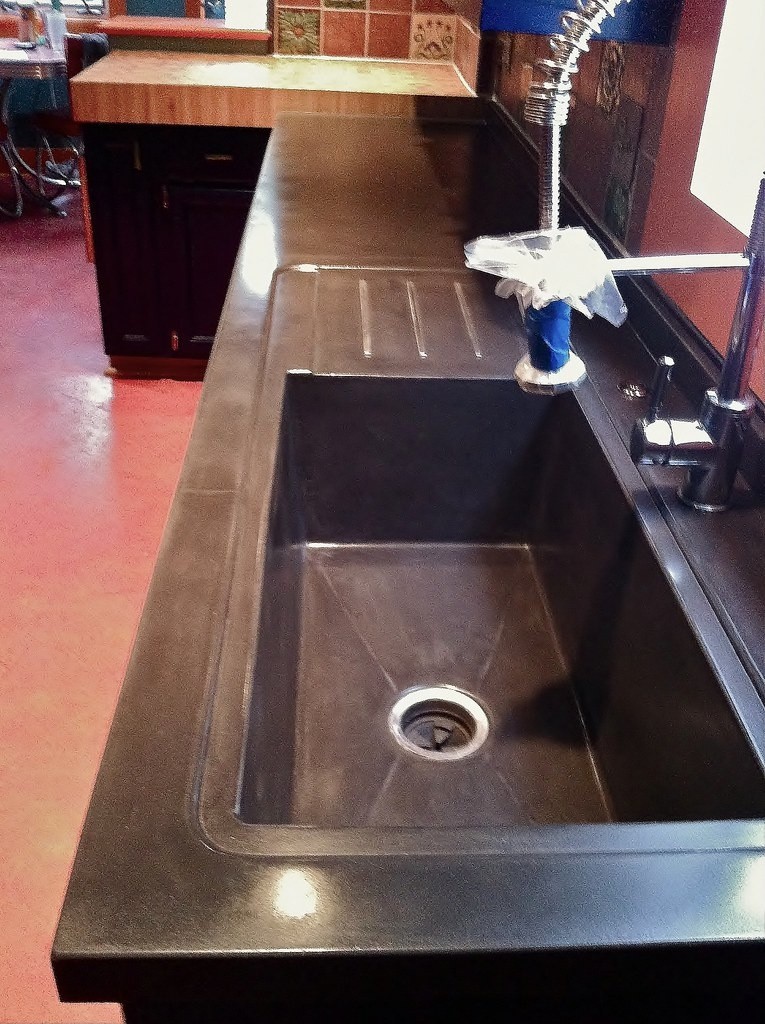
[72,118,271,384]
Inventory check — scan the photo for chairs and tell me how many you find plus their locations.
[14,33,110,206]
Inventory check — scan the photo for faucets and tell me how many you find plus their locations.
[510,266,595,397]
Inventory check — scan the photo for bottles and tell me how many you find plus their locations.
[47,0,67,50]
[19,12,34,42]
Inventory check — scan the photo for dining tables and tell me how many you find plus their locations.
[1,36,65,220]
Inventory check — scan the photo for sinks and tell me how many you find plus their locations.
[196,361,764,861]
